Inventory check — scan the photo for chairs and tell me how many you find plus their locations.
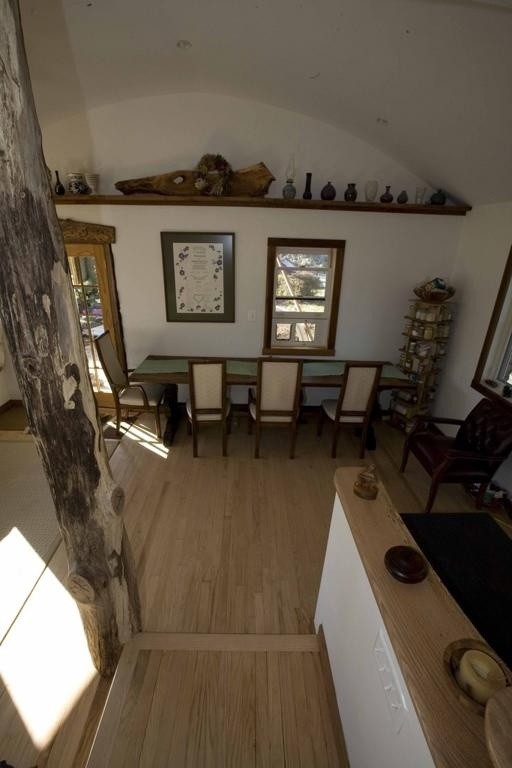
[400,398,512,513]
[248,358,304,459]
[184,359,231,457]
[94,330,165,438]
[317,362,383,458]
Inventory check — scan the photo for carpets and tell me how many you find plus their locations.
[0,405,139,645]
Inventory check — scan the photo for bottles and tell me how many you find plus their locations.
[394,307,452,416]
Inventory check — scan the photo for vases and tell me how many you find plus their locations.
[284,173,445,204]
[55,170,99,196]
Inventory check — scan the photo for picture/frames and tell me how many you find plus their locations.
[160,232,234,322]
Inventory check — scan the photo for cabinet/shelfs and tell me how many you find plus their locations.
[388,300,463,431]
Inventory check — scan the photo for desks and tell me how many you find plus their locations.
[129,356,417,450]
